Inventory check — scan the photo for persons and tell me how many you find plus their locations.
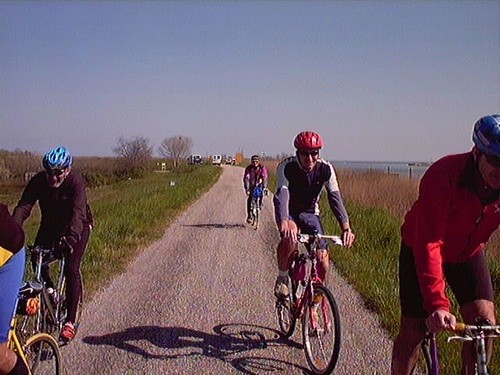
[0,204,28,375]
[391,115,500,375]
[11,145,93,341]
[273,131,355,336]
[244,156,268,223]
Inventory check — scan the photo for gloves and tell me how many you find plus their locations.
[53,238,71,260]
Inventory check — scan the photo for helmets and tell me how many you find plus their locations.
[294,131,322,150]
[41,145,73,171]
[472,114,500,159]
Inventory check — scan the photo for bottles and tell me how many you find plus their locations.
[46,287,59,305]
[295,281,306,298]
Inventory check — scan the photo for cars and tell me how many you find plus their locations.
[226,156,235,165]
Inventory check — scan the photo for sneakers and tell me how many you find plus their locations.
[61,320,76,342]
[49,290,57,306]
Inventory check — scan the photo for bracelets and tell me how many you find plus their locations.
[343,228,351,233]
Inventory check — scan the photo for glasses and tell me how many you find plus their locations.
[299,151,320,157]
[481,150,500,167]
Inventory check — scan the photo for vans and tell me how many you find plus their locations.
[212,154,221,164]
[191,154,201,165]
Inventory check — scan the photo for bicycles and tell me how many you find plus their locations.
[427,317,500,374]
[0,317,62,375]
[246,189,268,230]
[17,246,86,335]
[275,230,344,375]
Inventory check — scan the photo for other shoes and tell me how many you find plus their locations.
[247,216,252,223]
[274,275,289,299]
